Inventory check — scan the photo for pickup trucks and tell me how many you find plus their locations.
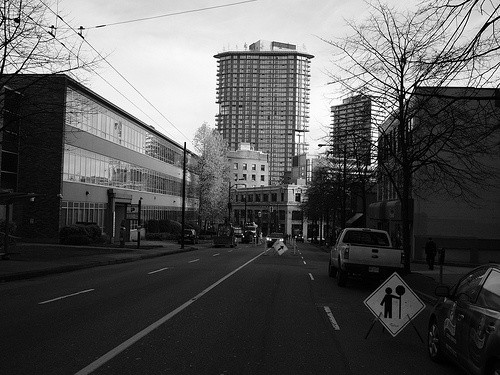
[325,226,406,291]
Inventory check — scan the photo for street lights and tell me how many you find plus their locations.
[318,144,347,228]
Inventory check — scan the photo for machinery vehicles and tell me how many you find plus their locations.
[212,223,238,248]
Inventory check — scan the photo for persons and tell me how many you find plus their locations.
[424,236,436,270]
[119,220,126,248]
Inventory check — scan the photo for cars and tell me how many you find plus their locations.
[267,233,286,248]
[427,263,500,375]
[178,229,199,245]
[295,234,304,243]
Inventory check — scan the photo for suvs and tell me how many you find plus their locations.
[243,226,258,244]
